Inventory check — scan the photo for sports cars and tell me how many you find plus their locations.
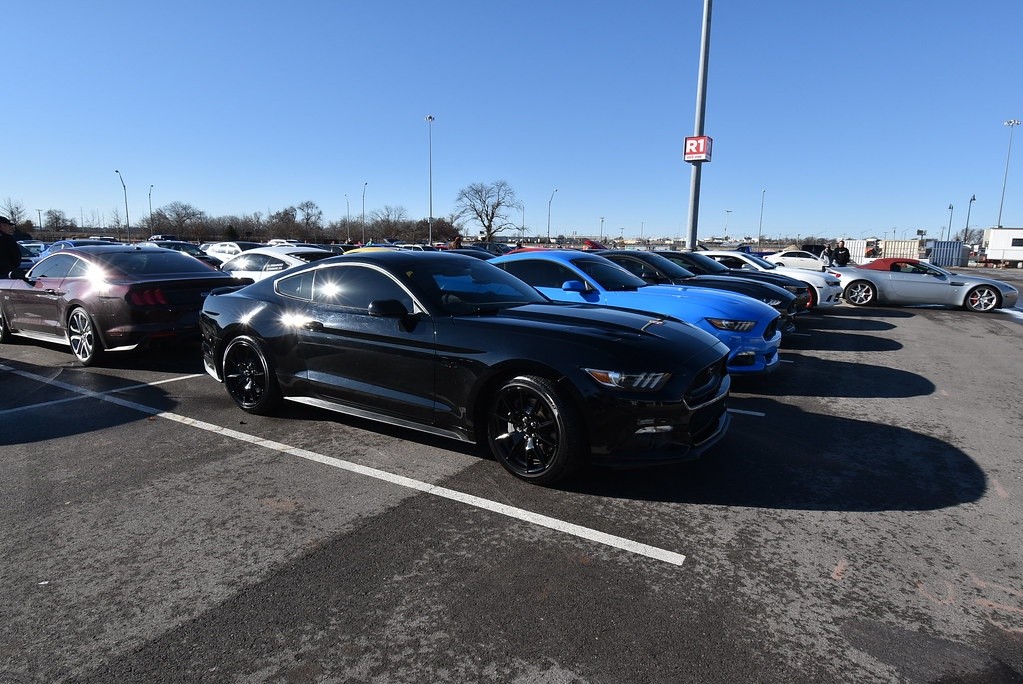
[826,258,1019,313]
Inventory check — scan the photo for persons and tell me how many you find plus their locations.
[0,216,21,278]
[449,236,463,249]
[820,240,850,267]
[366,237,375,246]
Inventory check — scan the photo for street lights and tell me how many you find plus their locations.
[946,194,976,243]
[363,182,368,244]
[345,194,349,239]
[149,184,153,236]
[425,116,435,244]
[758,190,766,252]
[997,120,1021,228]
[520,189,557,244]
[115,170,131,244]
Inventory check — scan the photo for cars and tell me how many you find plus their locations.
[216,246,338,286]
[652,251,811,315]
[8,243,41,279]
[435,249,782,374]
[0,245,243,366]
[595,251,797,335]
[200,250,734,483]
[16,235,512,260]
[694,252,844,314]
[761,250,826,272]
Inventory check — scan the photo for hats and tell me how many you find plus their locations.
[0,216,14,226]
